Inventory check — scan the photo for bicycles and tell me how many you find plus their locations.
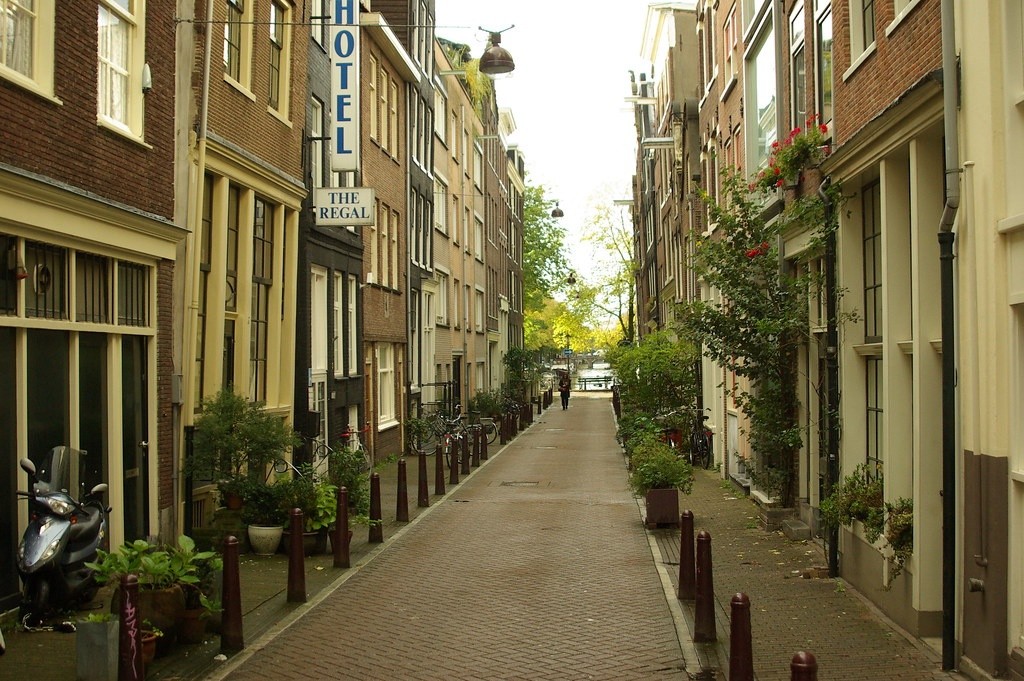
[339,421,373,480]
[411,400,498,469]
[661,407,713,470]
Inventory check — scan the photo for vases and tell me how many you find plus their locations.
[784,186,798,209]
[798,169,821,198]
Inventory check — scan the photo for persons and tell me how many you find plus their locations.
[558,375,571,410]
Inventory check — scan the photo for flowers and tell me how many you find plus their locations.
[782,113,829,170]
[748,142,796,194]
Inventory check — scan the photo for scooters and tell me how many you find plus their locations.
[14,445,113,631]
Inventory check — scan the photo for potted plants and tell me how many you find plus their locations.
[329,445,370,517]
[85,540,223,656]
[235,478,293,554]
[77,614,120,681]
[328,473,385,554]
[179,386,305,510]
[286,486,321,554]
[625,445,693,529]
[142,619,163,663]
[179,552,214,644]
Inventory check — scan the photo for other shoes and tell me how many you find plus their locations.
[563,407,565,410]
[566,406,567,409]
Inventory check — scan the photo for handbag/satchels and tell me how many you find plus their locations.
[558,387,568,392]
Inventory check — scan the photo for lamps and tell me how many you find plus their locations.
[174,17,514,74]
[508,268,576,284]
[432,191,565,217]
